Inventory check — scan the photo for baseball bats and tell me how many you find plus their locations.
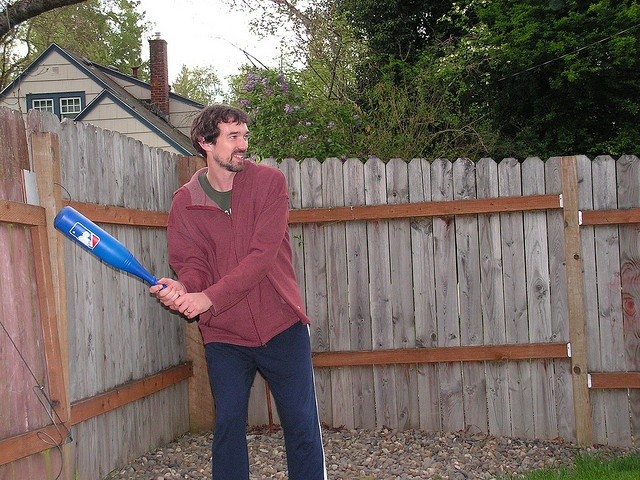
[53,205,181,298]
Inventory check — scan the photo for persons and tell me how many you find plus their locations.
[149,104,328,480]
